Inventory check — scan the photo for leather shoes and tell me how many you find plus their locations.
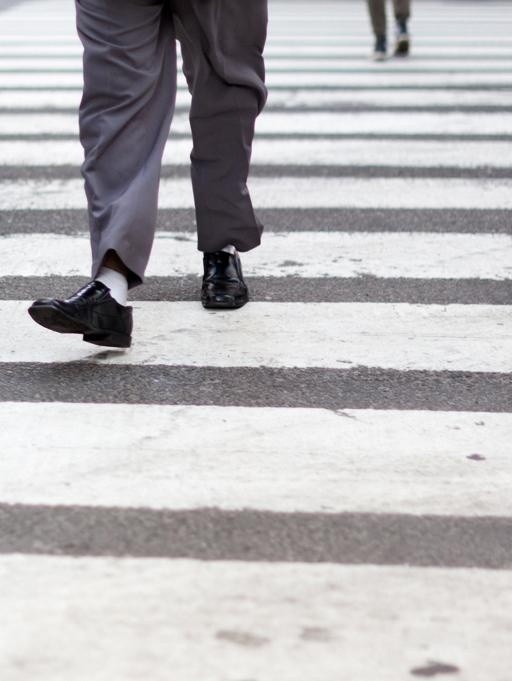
[28,279,133,348]
[202,249,248,309]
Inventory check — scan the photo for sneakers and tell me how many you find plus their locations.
[374,32,409,60]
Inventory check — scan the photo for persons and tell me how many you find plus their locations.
[366,1,411,63]
[26,1,272,352]
